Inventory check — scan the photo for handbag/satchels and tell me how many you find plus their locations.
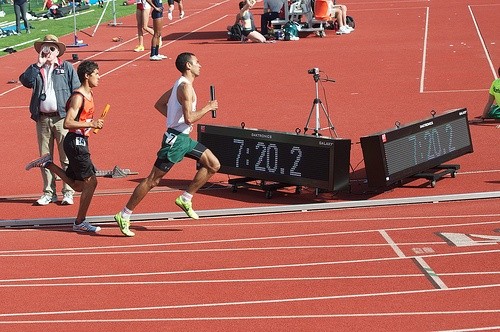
[346,16,355,28]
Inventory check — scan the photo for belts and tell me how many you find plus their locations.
[39,111,60,117]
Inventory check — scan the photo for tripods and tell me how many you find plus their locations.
[304,75,361,197]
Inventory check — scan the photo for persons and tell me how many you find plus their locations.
[10,0,31,35]
[19,34,81,206]
[259,0,354,35]
[227,0,267,44]
[25,60,105,233]
[115,53,221,238]
[42,0,89,12]
[146,0,167,61]
[133,0,162,52]
[98,0,104,8]
[123,0,140,6]
[167,0,184,20]
[472,68,500,128]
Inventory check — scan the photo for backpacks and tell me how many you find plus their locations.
[227,22,243,41]
[282,20,300,41]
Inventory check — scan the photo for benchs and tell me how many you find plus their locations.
[272,0,332,34]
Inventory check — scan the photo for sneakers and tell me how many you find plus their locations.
[26,153,52,171]
[34,191,58,205]
[72,218,102,233]
[113,211,135,237]
[175,195,200,221]
[61,190,74,205]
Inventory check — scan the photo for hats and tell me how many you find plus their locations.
[34,34,66,57]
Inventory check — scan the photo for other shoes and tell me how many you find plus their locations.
[168,10,173,21]
[68,9,72,15]
[26,29,31,34]
[75,11,80,14]
[179,12,184,19]
[15,33,22,36]
[159,36,163,48]
[338,26,350,34]
[345,25,355,32]
[134,47,145,52]
[240,34,246,44]
[98,5,103,8]
[149,54,168,60]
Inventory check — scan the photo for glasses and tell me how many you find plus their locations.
[50,47,59,51]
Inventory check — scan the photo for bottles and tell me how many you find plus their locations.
[43,46,50,57]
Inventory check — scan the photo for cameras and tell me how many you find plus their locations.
[308,68,324,74]
